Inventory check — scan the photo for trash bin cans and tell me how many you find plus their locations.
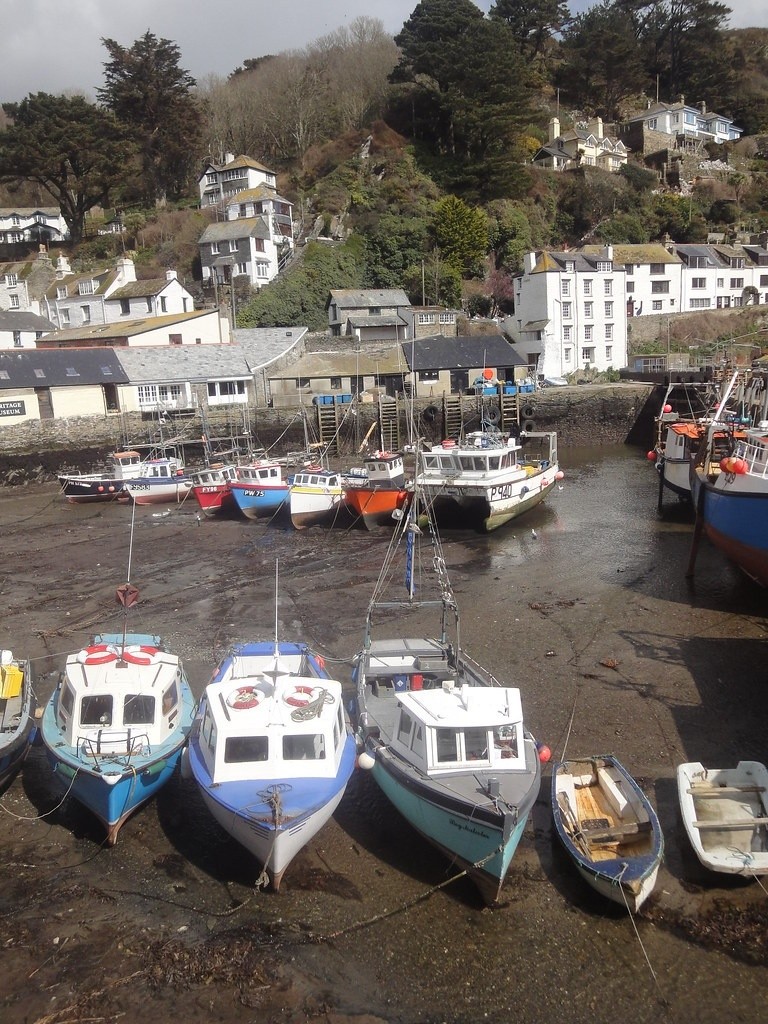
[314,394,353,404]
[474,385,533,395]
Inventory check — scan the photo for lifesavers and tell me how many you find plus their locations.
[124,645,163,666]
[283,685,320,708]
[425,405,438,418]
[521,404,536,419]
[306,466,323,473]
[484,405,501,425]
[522,420,537,432]
[76,643,120,665]
[227,685,267,710]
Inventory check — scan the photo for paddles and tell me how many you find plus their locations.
[557,790,595,863]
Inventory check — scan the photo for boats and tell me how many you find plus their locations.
[54,312,565,534]
[550,754,666,915]
[645,316,768,612]
[187,554,358,891]
[351,408,543,909]
[39,488,201,849]
[675,760,768,879]
[0,649,38,791]
